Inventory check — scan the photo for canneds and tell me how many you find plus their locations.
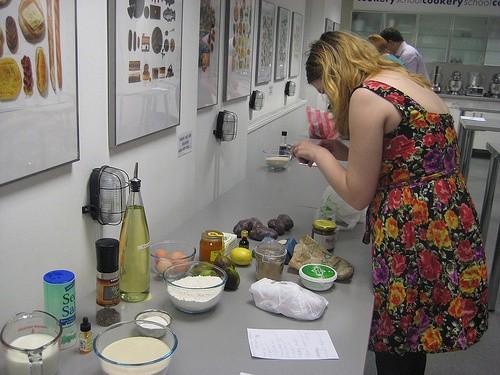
[199,229,223,264]
[311,219,337,253]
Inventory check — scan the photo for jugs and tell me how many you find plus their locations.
[0,310,63,375]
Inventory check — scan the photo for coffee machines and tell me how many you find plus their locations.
[448,71,462,94]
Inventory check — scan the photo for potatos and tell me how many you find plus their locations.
[233,214,295,241]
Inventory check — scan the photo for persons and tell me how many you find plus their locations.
[366,35,407,67]
[291,31,489,375]
[381,28,430,84]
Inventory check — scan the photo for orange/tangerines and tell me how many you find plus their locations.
[155,248,192,274]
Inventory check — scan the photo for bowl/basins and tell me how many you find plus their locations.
[263,150,292,171]
[299,263,338,292]
[449,80,461,91]
[162,261,228,314]
[149,240,196,281]
[93,319,178,375]
[134,309,174,339]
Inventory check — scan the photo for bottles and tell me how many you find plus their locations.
[279,130,288,158]
[94,238,121,327]
[79,316,94,354]
[119,161,151,303]
[239,230,249,250]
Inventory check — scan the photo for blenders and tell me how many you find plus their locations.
[431,67,442,92]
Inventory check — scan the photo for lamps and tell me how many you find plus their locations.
[285,81,296,96]
[82,165,131,226]
[249,89,265,111]
[213,109,239,142]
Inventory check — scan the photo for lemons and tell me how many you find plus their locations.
[230,247,254,265]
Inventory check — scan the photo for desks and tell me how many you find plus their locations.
[451,100,500,137]
[479,142,500,314]
[458,113,500,185]
[0,133,376,375]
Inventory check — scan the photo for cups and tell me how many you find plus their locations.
[252,240,287,282]
[43,270,77,350]
